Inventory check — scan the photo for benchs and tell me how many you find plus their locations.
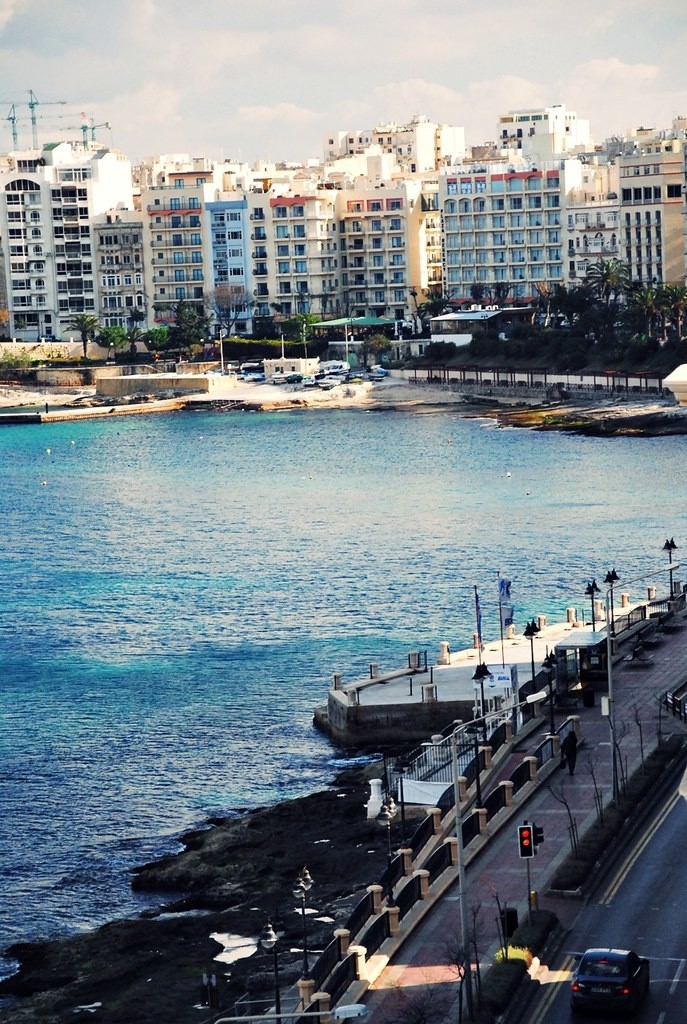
[621,609,680,669]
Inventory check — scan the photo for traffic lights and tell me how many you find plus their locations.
[533,823,545,855]
[518,826,534,859]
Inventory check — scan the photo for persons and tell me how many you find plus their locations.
[562,731,578,775]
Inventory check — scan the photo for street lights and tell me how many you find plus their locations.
[663,538,678,601]
[523,618,541,694]
[374,797,397,907]
[464,714,486,809]
[258,918,281,1024]
[472,662,491,746]
[452,692,547,1024]
[603,568,619,637]
[584,579,601,633]
[541,653,559,736]
[606,562,680,804]
[392,756,411,849]
[293,866,316,980]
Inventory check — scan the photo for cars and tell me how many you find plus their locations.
[571,947,650,1018]
[239,359,350,383]
[37,333,217,363]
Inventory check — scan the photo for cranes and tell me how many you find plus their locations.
[0,90,111,151]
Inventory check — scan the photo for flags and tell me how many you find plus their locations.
[502,607,514,628]
[476,594,485,652]
[499,578,511,601]
